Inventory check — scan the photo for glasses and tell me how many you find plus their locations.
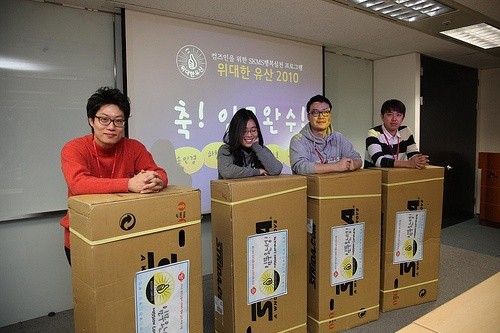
[243,127,259,134]
[95,115,126,127]
[309,109,331,117]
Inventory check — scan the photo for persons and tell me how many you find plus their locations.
[363,98,431,170]
[289,94,363,176]
[217,108,284,179]
[60,86,168,266]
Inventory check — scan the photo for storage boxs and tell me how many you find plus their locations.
[299,169,382,333]
[211,174,307,333]
[68,185,204,333]
[368,165,444,312]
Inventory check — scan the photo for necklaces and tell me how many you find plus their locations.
[92,141,118,181]
[384,134,400,161]
